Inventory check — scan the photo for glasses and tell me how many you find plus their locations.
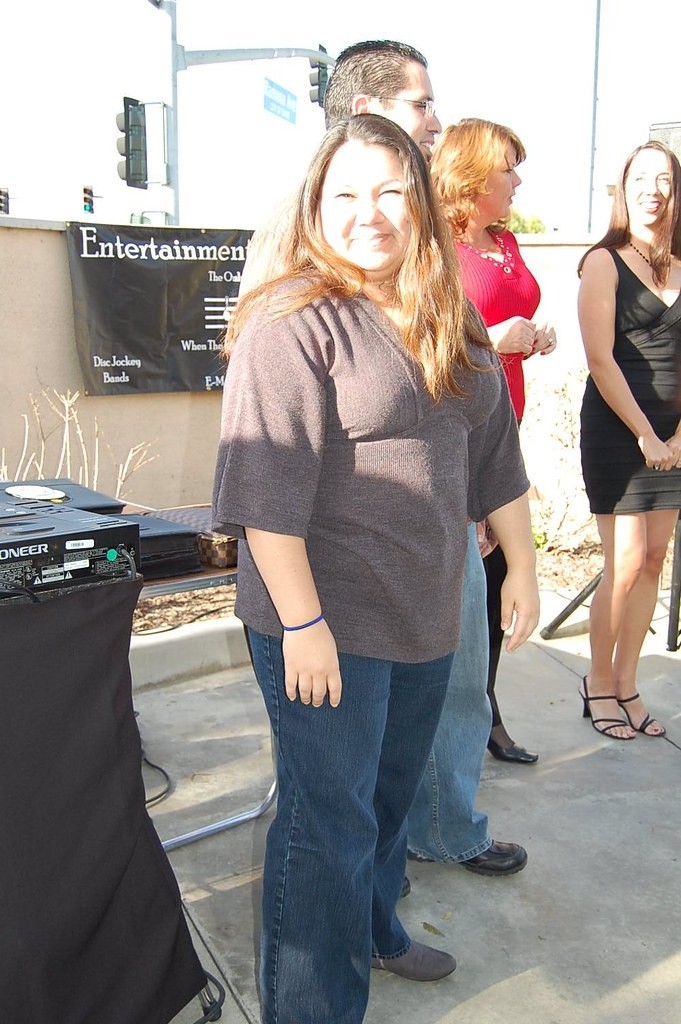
[370,95,437,117]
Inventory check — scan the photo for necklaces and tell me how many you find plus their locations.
[628,241,656,270]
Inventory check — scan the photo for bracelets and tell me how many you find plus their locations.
[282,613,324,633]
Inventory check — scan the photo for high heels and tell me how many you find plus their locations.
[487,738,538,763]
[578,675,666,741]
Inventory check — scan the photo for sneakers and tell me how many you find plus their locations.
[371,940,457,982]
[406,837,528,877]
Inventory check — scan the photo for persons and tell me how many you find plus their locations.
[434,117,565,766]
[573,137,681,742]
[207,108,546,1020]
[234,37,532,882]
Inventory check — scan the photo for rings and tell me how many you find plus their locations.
[655,464,660,470]
